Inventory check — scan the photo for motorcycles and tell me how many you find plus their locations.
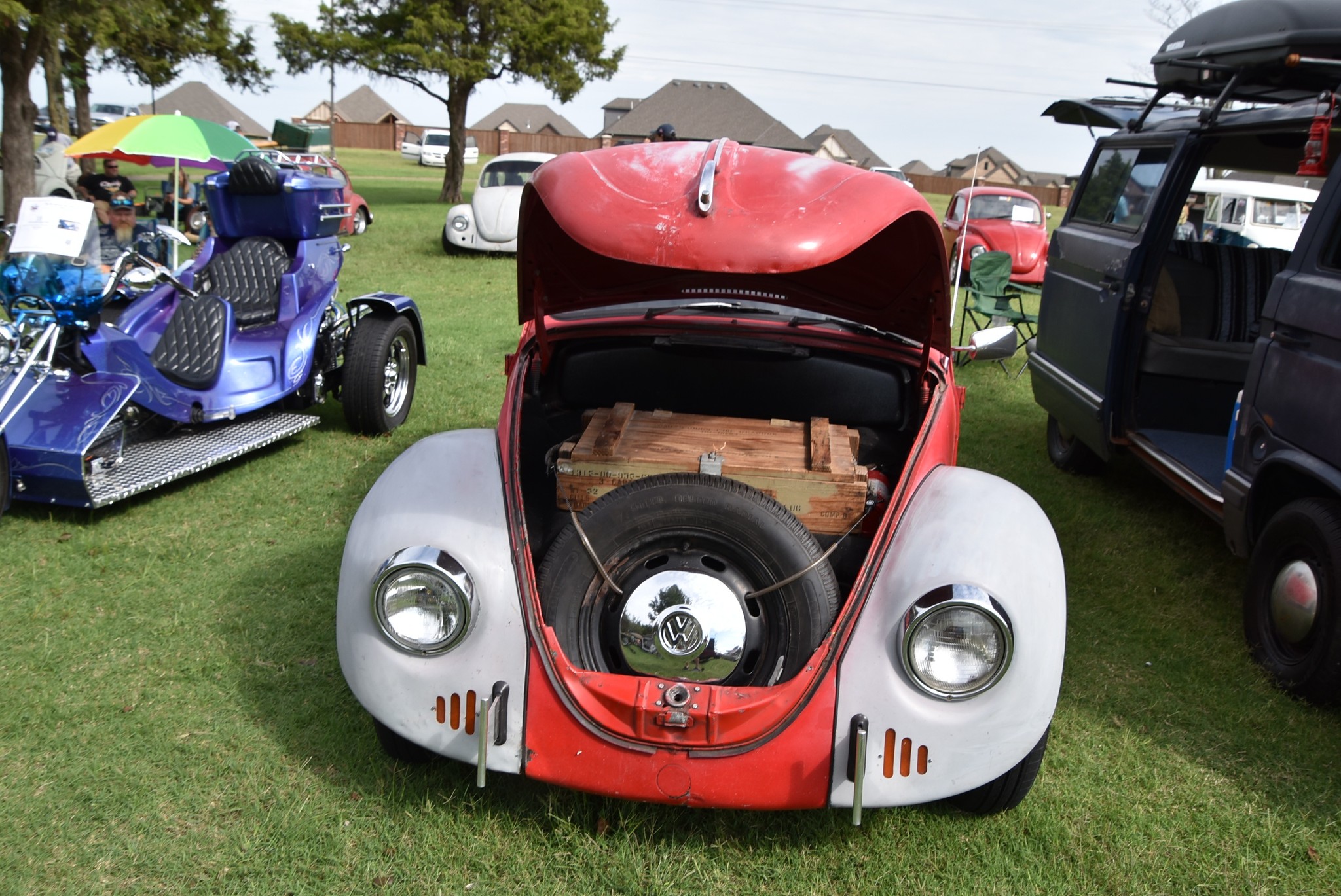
[0,149,428,522]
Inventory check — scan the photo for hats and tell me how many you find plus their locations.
[110,191,133,211]
[650,123,675,138]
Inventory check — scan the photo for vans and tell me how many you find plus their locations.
[1025,0,1341,711]
[401,128,479,168]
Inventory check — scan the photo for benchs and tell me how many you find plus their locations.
[1140,238,1292,384]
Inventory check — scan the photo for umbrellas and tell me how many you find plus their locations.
[62,109,260,270]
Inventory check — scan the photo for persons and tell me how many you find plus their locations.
[86,159,138,226]
[643,124,676,143]
[164,166,197,227]
[86,191,162,276]
[39,126,83,196]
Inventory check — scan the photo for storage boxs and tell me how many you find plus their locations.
[1147,0,1341,95]
[554,400,872,538]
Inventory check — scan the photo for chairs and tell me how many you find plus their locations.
[955,250,1038,382]
[197,236,293,333]
[146,295,227,386]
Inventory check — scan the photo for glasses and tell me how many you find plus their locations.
[111,198,133,207]
[105,165,118,169]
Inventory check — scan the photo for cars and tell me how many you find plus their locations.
[184,153,374,246]
[0,127,79,222]
[441,152,559,259]
[867,167,914,188]
[72,102,145,139]
[939,186,1053,287]
[337,136,1066,825]
[33,104,79,137]
[1191,179,1321,252]
